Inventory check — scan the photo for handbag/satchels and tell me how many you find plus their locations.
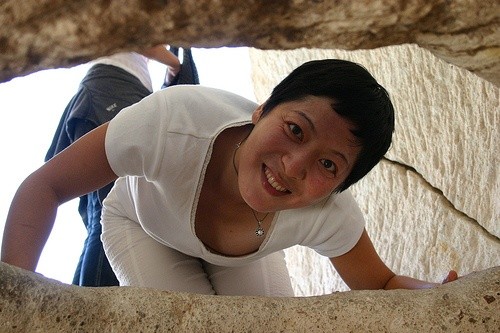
[162,46,201,88]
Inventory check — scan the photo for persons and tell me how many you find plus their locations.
[42,43,182,287]
[2,59,459,297]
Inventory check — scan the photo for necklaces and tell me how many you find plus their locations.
[232,136,273,237]
